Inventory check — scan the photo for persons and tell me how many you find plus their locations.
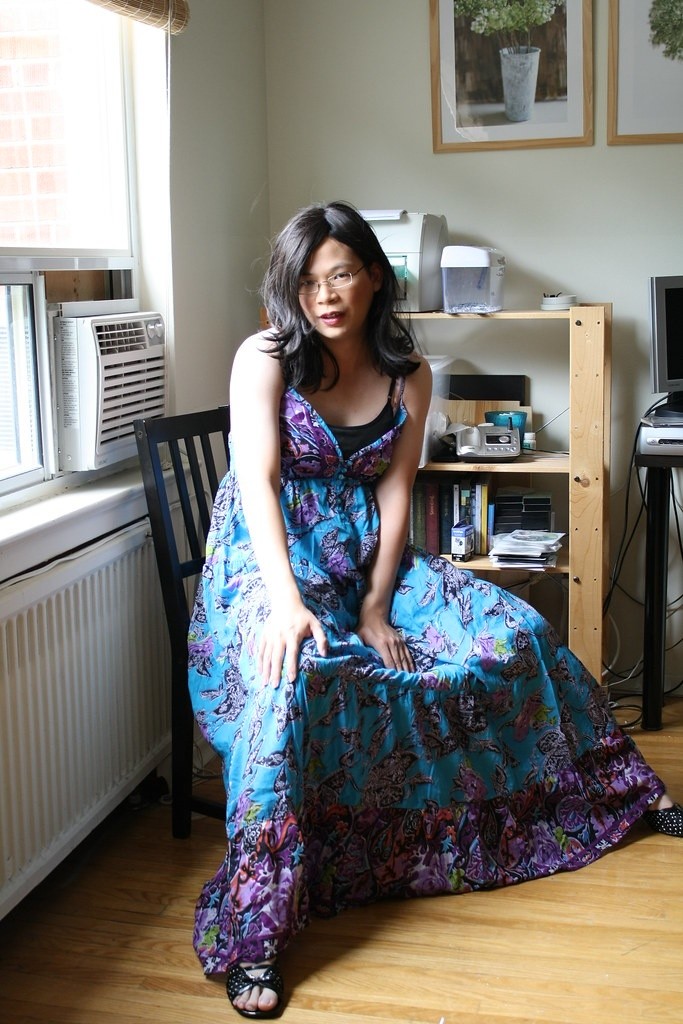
[183,198,683,1019]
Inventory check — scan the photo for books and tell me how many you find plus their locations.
[412,478,567,571]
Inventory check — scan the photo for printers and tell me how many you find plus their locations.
[357,209,448,313]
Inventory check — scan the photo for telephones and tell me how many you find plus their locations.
[455,417,521,463]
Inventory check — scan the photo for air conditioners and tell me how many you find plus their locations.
[54,309,167,471]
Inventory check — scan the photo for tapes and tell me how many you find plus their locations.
[540,295,579,311]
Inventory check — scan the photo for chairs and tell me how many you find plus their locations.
[131,405,233,842]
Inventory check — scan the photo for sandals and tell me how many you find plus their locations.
[634,804,683,838]
[227,961,285,1019]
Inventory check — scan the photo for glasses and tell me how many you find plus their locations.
[297,265,365,294]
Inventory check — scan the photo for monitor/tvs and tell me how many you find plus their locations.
[651,276,683,417]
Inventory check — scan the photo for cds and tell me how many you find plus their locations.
[513,533,557,541]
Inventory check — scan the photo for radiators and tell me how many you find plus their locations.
[0,457,213,922]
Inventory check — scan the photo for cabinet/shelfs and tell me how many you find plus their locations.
[260,301,613,706]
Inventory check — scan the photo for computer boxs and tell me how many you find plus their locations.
[640,416,683,457]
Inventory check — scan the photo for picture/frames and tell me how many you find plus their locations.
[606,0,683,147]
[427,1,594,155]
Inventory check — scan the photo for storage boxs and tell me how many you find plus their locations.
[438,244,507,314]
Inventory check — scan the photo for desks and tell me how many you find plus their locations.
[633,446,683,733]
